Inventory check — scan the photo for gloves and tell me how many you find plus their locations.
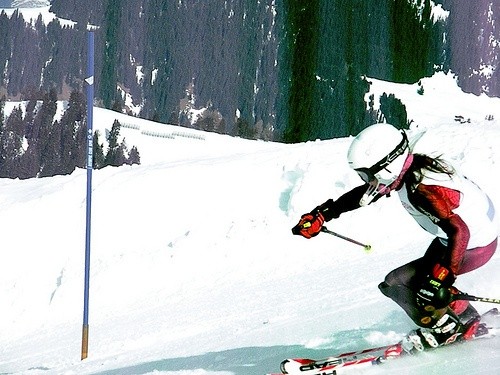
[417,263,455,307]
[298,205,325,238]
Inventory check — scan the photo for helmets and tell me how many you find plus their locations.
[348,123,410,207]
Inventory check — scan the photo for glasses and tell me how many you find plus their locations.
[353,132,408,184]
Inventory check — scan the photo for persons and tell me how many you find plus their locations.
[291,123,498,350]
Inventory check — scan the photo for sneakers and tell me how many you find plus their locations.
[400,304,480,351]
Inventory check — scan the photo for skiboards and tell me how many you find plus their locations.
[281,308,500,375]
